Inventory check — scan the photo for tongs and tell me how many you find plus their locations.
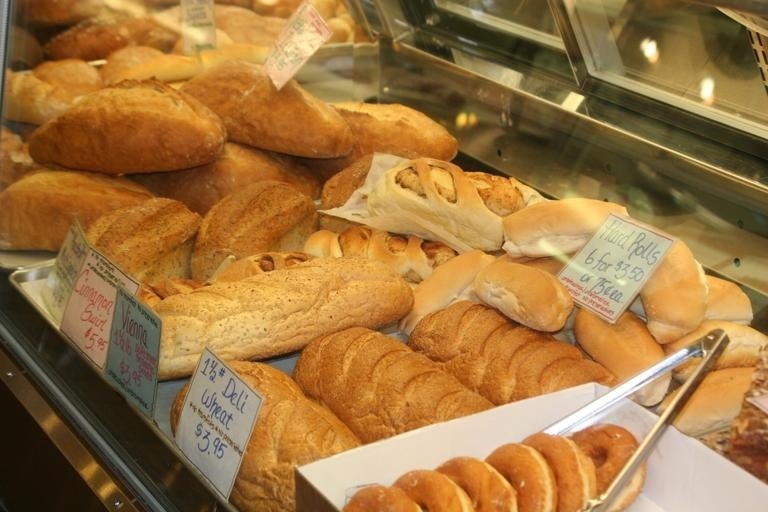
[542,328,732,512]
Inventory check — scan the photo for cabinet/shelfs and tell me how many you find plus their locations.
[0,0,768,512]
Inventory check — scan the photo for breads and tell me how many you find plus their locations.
[1,2,767,510]
